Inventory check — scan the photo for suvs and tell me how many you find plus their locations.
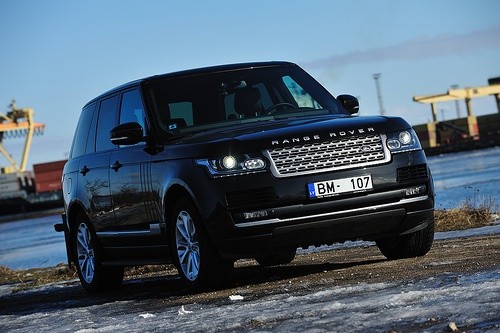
[53,60,437,295]
